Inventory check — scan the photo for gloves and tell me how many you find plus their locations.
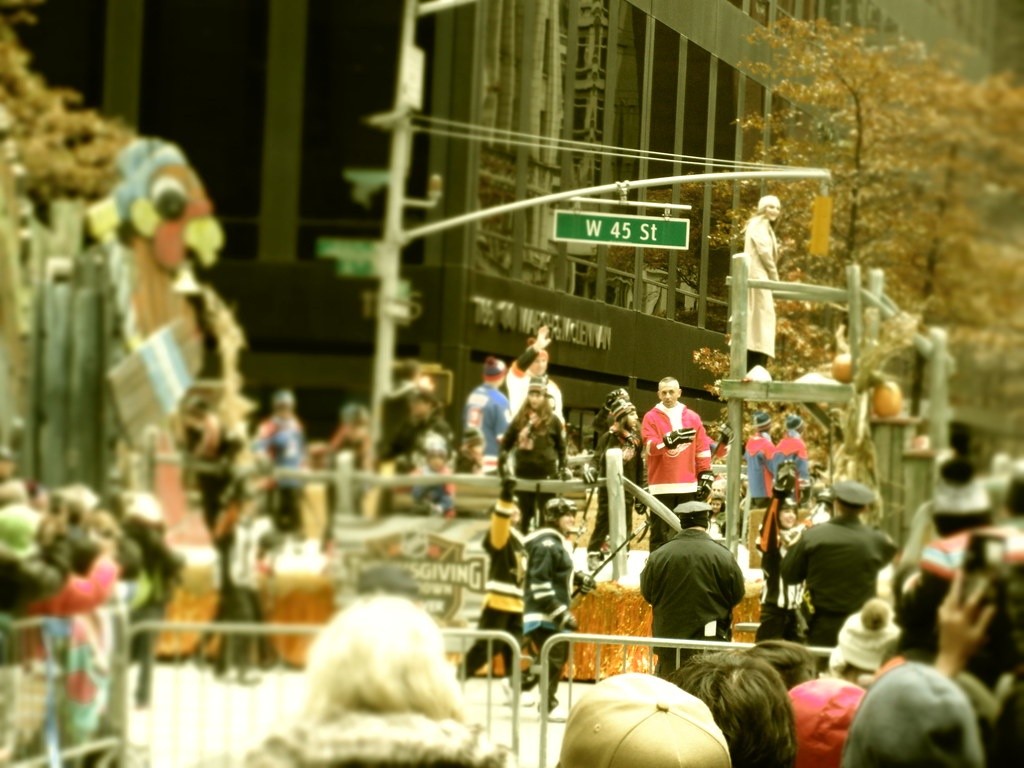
[558,466,573,481]
[582,467,600,484]
[694,470,715,502]
[663,426,696,448]
[555,611,580,631]
[634,497,648,515]
[499,476,517,502]
[575,571,597,595]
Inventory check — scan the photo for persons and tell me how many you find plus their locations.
[232,592,513,768]
[728,195,781,373]
[743,412,807,561]
[182,322,454,691]
[458,326,568,713]
[0,449,183,768]
[585,377,716,571]
[562,446,1024,768]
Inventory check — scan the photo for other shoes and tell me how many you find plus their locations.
[539,706,568,722]
[500,679,516,702]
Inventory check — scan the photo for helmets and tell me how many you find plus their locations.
[546,497,578,528]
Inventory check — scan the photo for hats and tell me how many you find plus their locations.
[752,410,772,429]
[785,411,803,431]
[832,480,875,511]
[556,672,731,768]
[528,376,549,393]
[674,500,713,529]
[481,356,508,382]
[526,338,549,361]
[461,427,484,448]
[838,599,902,672]
[604,387,637,422]
